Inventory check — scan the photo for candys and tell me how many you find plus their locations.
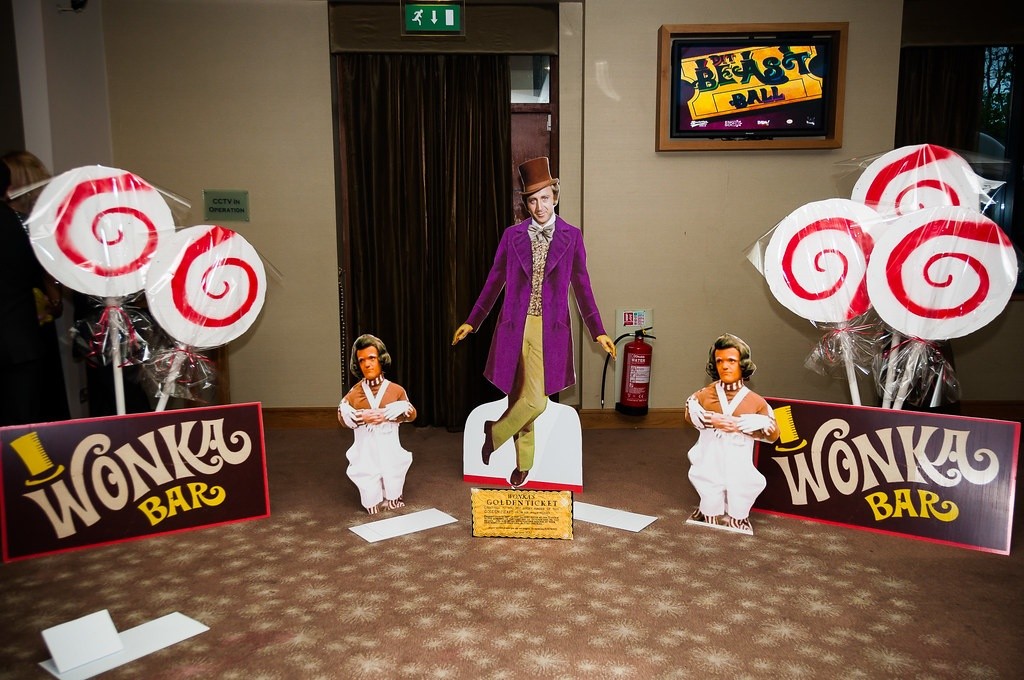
[850,142,982,409]
[763,199,886,406]
[144,225,266,412]
[26,163,176,416]
[865,205,1019,412]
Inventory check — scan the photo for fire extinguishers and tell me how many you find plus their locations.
[601,326,656,415]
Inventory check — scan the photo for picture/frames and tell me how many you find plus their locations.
[659,22,849,150]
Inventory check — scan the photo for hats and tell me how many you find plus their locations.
[513,156,560,196]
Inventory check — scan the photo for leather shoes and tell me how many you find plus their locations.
[510,465,530,485]
[481,420,495,465]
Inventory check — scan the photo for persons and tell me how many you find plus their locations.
[338,334,417,516]
[0,150,71,428]
[453,156,616,488]
[685,333,781,529]
